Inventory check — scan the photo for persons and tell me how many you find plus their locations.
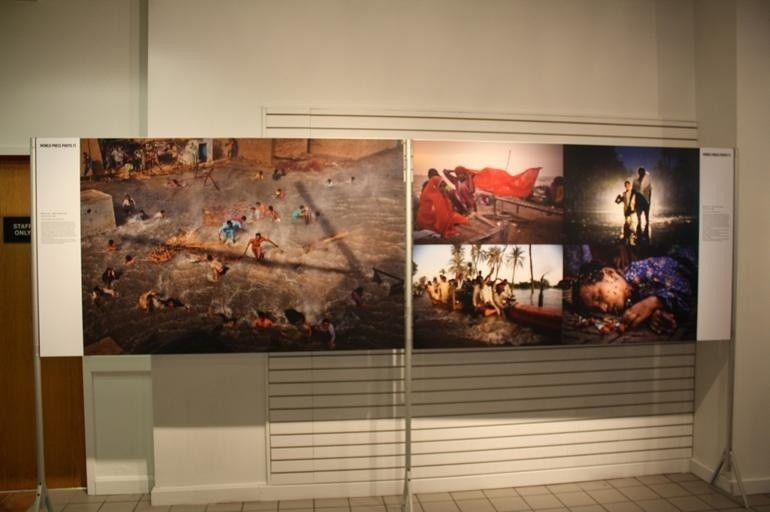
[569,256,697,334]
[615,166,653,247]
[416,167,543,237]
[427,275,512,316]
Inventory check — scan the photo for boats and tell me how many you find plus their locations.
[427,285,562,335]
